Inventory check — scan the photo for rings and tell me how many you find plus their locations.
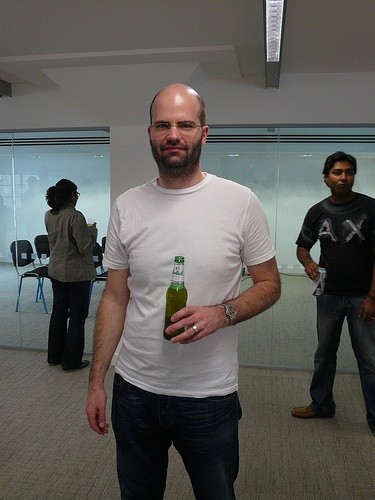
[192,325,199,334]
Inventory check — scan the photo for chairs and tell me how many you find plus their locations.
[92,236,108,283]
[34,235,55,299]
[9,239,51,315]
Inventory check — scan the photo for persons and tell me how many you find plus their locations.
[292,151,375,435]
[86,84,281,500]
[45,179,98,372]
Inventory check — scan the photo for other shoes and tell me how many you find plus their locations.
[49,359,90,373]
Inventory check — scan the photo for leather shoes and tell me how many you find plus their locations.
[291,405,335,418]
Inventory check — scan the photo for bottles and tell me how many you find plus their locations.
[162,255,188,340]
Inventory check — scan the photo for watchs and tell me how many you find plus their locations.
[222,303,238,326]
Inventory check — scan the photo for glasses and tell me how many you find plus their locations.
[150,122,203,131]
[74,192,81,196]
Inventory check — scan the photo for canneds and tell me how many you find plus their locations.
[312,268,326,296]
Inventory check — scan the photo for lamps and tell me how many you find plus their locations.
[261,0,286,89]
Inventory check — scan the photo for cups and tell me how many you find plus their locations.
[87,218,95,226]
